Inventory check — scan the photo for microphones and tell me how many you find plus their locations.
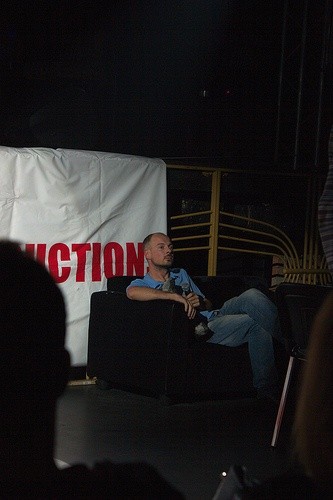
[181,283,191,296]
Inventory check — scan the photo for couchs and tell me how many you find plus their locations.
[87,277,267,407]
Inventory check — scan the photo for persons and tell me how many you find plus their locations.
[0,242,184,500]
[126,233,309,403]
[213,293,333,500]
